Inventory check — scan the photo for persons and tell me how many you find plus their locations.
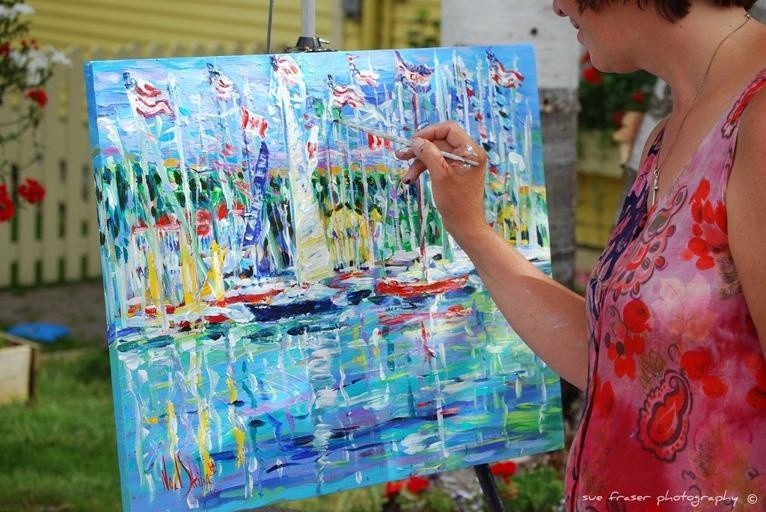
[393,0,766,512]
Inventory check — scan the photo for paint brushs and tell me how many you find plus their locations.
[330,117,483,167]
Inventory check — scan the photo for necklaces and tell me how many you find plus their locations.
[652,14,751,206]
